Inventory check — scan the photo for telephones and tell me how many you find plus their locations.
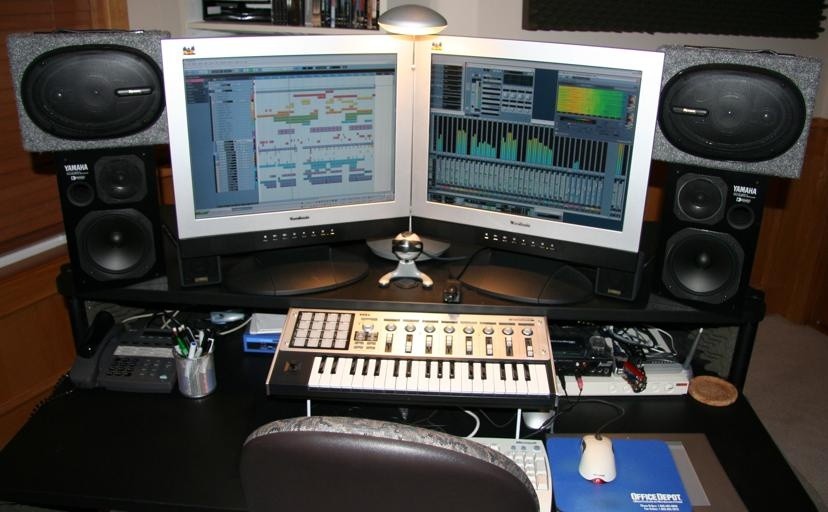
[70,310,178,393]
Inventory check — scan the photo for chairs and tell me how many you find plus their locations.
[236,420,542,512]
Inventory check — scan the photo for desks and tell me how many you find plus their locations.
[2,249,819,511]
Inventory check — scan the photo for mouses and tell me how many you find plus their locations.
[579,435,618,484]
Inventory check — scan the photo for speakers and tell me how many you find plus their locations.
[5,28,170,155]
[54,146,167,288]
[592,245,652,310]
[653,163,765,317]
[651,43,822,180]
[160,219,226,295]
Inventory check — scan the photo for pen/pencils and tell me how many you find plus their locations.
[172,324,215,396]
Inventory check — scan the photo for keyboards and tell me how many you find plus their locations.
[466,436,554,512]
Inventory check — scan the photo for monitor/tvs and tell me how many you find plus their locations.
[410,34,665,306]
[159,32,410,298]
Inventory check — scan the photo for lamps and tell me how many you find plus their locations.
[378,4,448,36]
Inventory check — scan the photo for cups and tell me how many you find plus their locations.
[172,347,216,399]
